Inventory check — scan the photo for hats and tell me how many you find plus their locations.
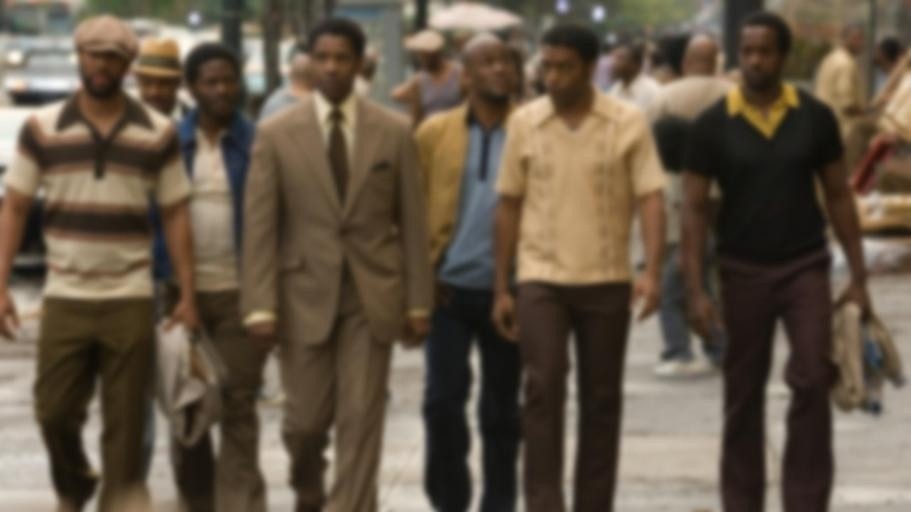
[73,14,139,63]
[132,36,184,78]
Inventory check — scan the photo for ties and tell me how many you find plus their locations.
[326,107,352,207]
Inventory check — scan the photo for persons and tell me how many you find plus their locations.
[154,43,267,512]
[261,16,911,381]
[682,15,872,512]
[490,21,667,512]
[240,17,433,512]
[1,13,203,511]
[133,33,192,128]
[410,33,539,512]
